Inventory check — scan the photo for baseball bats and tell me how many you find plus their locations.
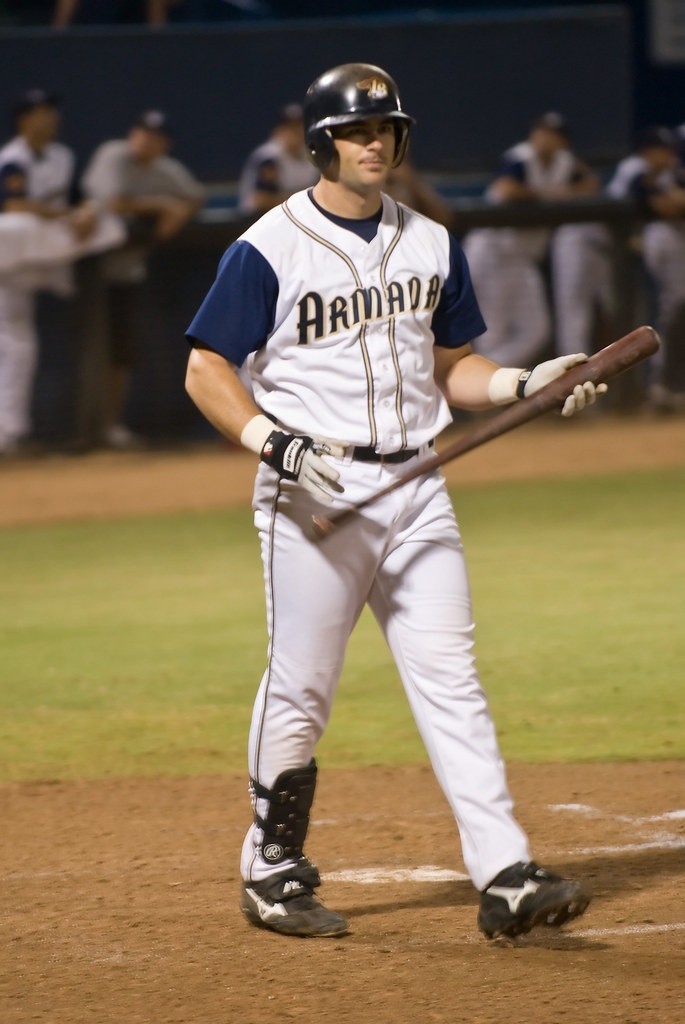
[310,323,662,540]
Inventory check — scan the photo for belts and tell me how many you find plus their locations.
[350,444,417,463]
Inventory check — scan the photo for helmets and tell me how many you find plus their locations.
[302,61,407,175]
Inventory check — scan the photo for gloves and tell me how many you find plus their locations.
[489,351,609,421]
[260,425,346,509]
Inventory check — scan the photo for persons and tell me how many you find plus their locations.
[184,62,609,944]
[0,87,685,468]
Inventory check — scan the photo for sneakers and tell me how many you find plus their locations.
[242,868,350,940]
[478,858,592,944]
